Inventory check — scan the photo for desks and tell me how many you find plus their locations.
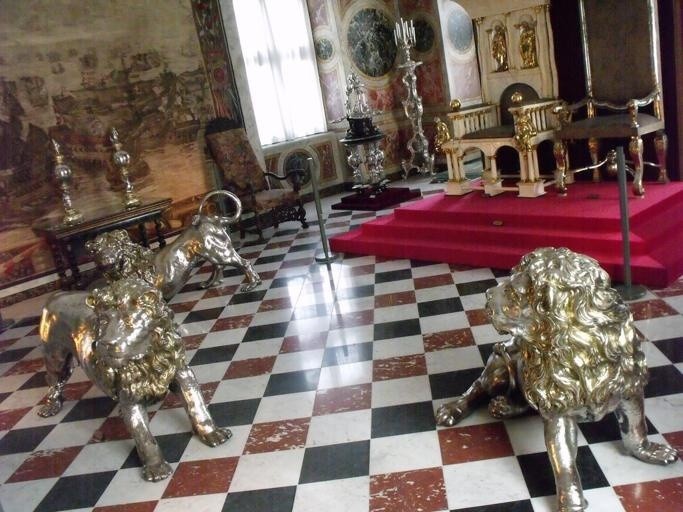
[31,196,173,289]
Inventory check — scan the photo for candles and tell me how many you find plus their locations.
[394,18,416,46]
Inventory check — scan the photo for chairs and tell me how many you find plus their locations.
[441,1,575,198]
[551,1,669,199]
[205,118,309,242]
